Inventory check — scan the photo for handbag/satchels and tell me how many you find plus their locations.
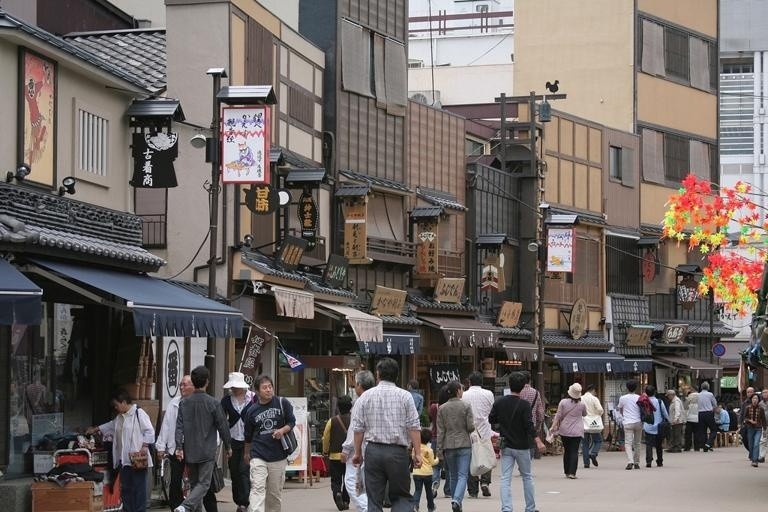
[210,462,224,493]
[279,396,297,455]
[658,421,672,435]
[128,452,148,470]
[470,424,497,477]
[495,437,504,450]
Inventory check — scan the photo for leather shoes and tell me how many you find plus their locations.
[626,447,713,469]
[344,501,349,510]
[591,455,598,466]
[452,502,461,512]
[335,493,344,511]
[237,505,246,512]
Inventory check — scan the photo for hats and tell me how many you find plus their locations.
[222,372,249,389]
[567,382,582,400]
[664,390,675,397]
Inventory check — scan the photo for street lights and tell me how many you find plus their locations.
[193,66,227,403]
[527,157,549,415]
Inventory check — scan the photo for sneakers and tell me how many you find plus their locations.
[568,473,576,478]
[431,481,439,498]
[481,483,491,496]
[174,505,185,512]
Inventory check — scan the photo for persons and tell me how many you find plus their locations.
[551,379,768,479]
[322,357,546,512]
[84,365,296,512]
[25,52,53,167]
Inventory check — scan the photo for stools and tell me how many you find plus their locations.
[706,421,742,449]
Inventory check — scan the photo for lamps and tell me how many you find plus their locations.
[5,161,31,185]
[54,175,77,201]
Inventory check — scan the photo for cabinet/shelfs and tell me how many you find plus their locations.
[306,380,331,432]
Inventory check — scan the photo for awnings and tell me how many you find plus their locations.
[664,357,724,369]
[546,350,653,373]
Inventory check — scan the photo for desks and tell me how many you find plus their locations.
[299,452,329,483]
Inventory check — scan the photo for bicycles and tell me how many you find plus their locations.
[575,433,594,456]
[161,453,188,506]
